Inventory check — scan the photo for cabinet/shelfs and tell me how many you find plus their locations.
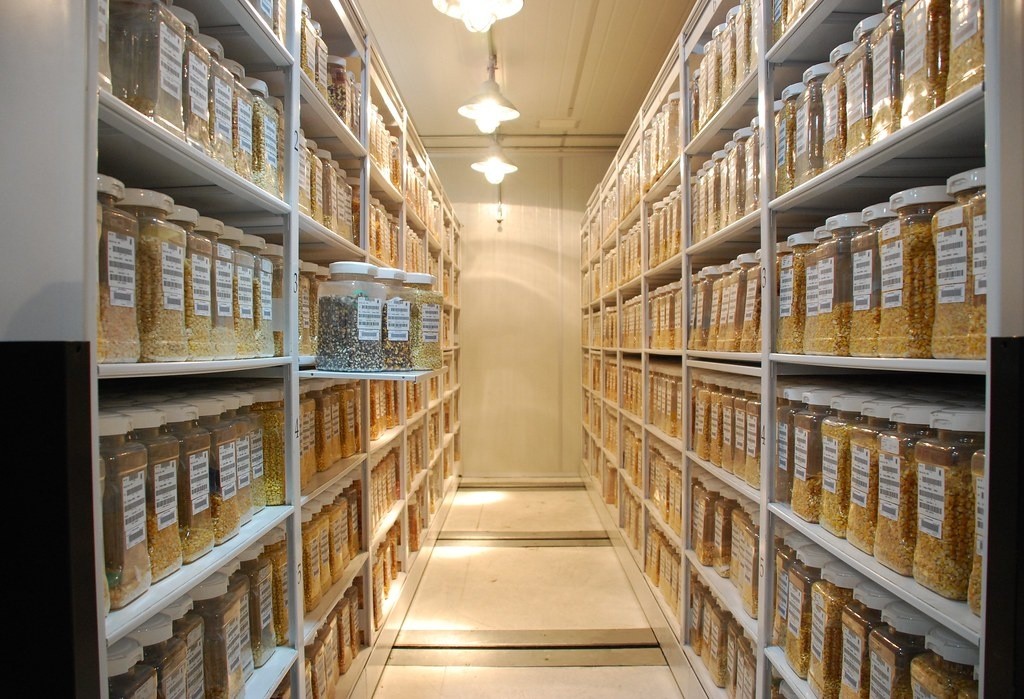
[579,0,1024,699]
[0,0,465,699]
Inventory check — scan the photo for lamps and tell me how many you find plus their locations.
[458,26,521,136]
[469,127,518,186]
